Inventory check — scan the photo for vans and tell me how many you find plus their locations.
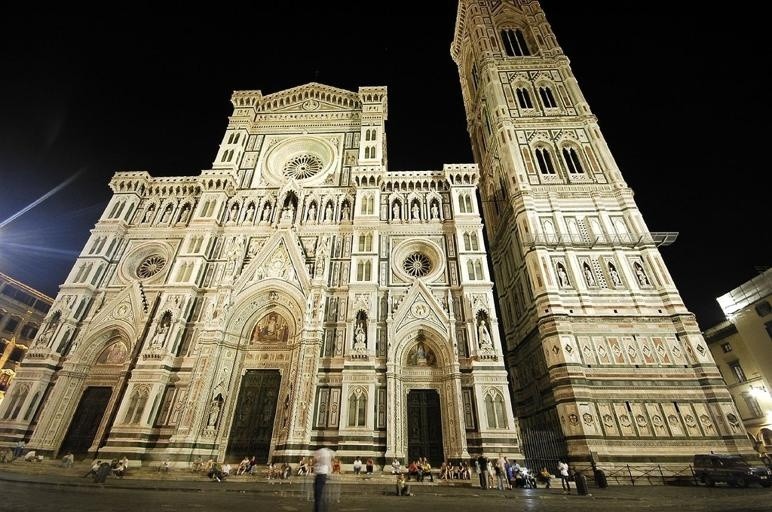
[693,453,771,487]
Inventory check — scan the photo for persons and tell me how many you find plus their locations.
[158,455,170,474]
[266,442,342,512]
[365,456,374,474]
[268,315,276,334]
[390,450,572,496]
[13,439,26,457]
[191,454,257,482]
[207,400,219,426]
[35,321,57,349]
[558,264,648,287]
[353,328,366,351]
[59,450,74,467]
[84,455,129,485]
[478,319,494,350]
[353,456,362,475]
[25,449,38,462]
[151,320,170,349]
[145,201,440,225]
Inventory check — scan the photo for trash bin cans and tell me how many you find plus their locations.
[574,472,588,496]
[94,462,110,483]
[596,470,609,488]
